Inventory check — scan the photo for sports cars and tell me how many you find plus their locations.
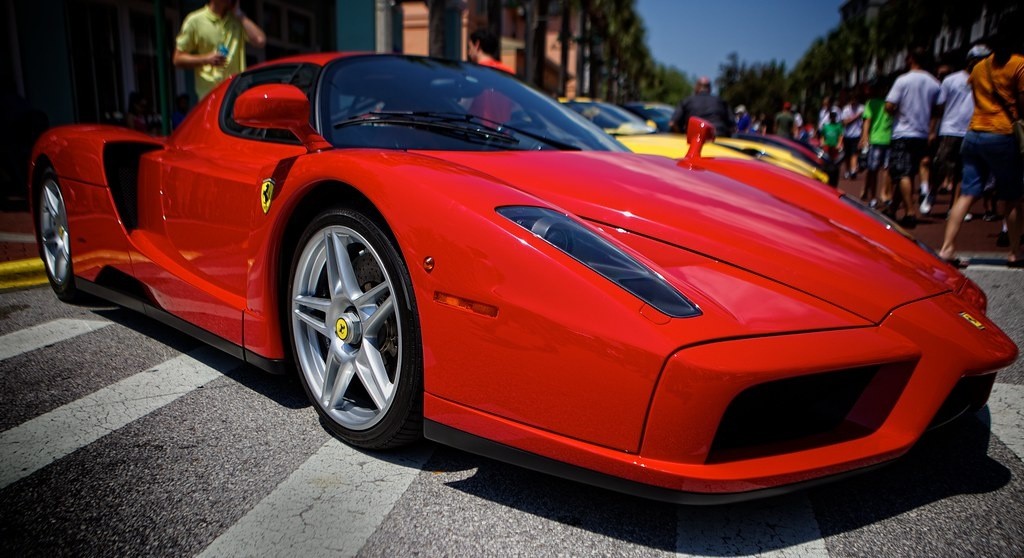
[33,48,1022,508]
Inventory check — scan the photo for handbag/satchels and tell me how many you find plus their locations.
[1012,119,1024,152]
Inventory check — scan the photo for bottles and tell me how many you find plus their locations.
[217,40,228,68]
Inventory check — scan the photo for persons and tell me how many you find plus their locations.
[774,52,941,218]
[669,77,728,136]
[736,106,767,136]
[127,92,189,134]
[468,31,517,134]
[918,44,991,220]
[174,0,265,99]
[938,7,1024,268]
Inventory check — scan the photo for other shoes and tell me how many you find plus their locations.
[860,193,869,202]
[938,252,968,268]
[869,198,879,210]
[946,209,972,221]
[903,214,917,228]
[920,191,936,214]
[996,231,1010,247]
[881,208,897,222]
[848,168,857,180]
[983,211,1005,221]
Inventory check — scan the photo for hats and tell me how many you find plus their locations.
[784,101,792,109]
[734,105,746,114]
[967,45,992,59]
